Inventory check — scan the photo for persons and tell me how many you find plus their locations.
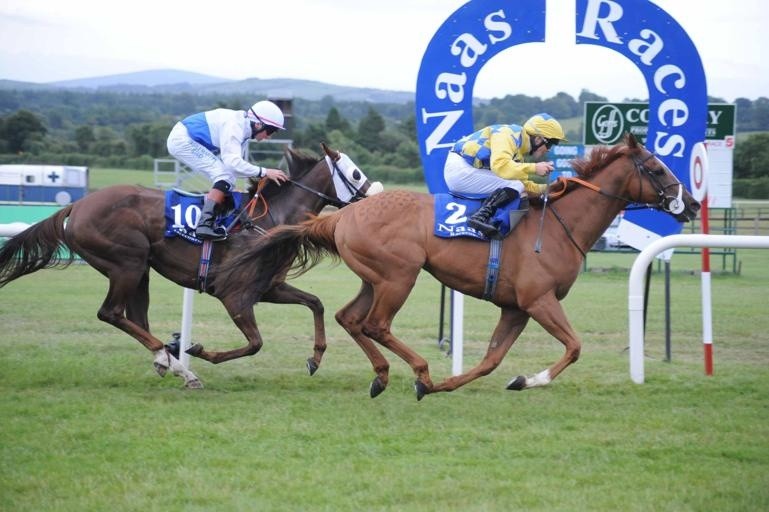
[442,112,568,241]
[165,99,290,242]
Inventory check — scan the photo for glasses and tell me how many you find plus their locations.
[249,106,279,135]
[529,119,553,150]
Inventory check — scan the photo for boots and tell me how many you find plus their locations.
[467,188,512,241]
[196,199,224,239]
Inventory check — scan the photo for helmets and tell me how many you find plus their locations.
[248,101,285,131]
[523,113,568,142]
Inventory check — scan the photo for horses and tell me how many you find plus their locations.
[0,138,387,393]
[215,127,703,403]
